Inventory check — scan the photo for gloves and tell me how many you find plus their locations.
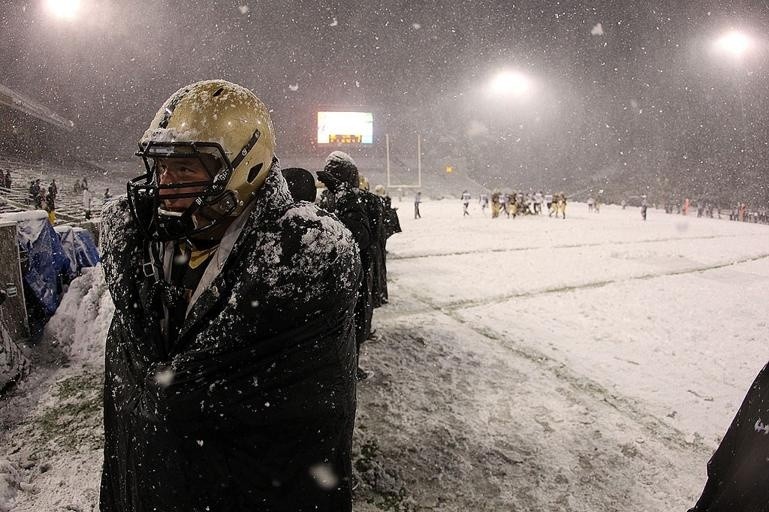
[317,171,340,193]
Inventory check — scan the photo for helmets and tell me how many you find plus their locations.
[127,80,275,242]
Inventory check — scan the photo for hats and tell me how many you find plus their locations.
[281,168,316,204]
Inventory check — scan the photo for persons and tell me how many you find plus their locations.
[99,79,362,512]
[586,193,628,214]
[640,195,648,220]
[0,168,113,226]
[414,192,423,219]
[461,190,471,216]
[478,191,567,219]
[280,150,402,386]
[663,191,769,225]
